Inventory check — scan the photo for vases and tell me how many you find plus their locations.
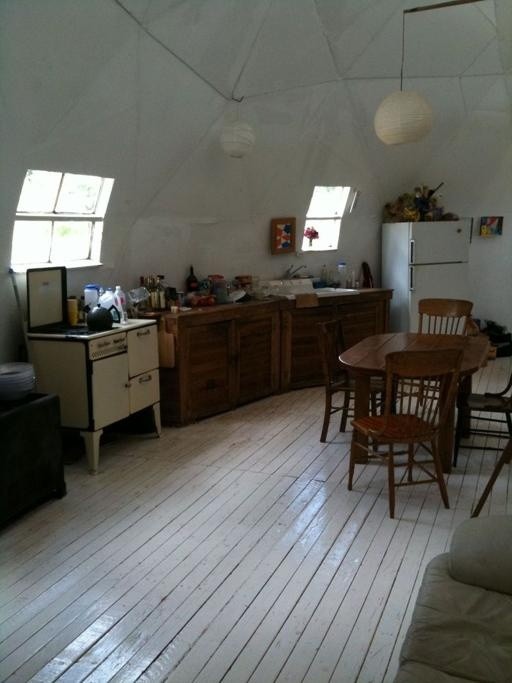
[309,237,313,247]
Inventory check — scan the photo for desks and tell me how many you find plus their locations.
[281,288,396,393]
[339,331,491,474]
[137,297,281,427]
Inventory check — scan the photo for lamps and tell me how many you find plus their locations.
[374,9,436,146]
[218,91,257,160]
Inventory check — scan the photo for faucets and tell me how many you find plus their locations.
[286,264,307,280]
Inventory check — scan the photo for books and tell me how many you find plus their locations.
[274,277,359,298]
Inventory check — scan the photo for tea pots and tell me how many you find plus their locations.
[82,303,121,331]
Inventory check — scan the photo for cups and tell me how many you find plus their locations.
[176,291,184,305]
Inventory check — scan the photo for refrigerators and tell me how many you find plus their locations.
[381,219,471,335]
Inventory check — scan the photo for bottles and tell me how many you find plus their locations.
[85,283,126,320]
[185,263,198,290]
[146,274,166,312]
[320,263,328,279]
[338,261,347,287]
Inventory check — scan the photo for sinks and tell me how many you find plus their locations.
[315,288,358,294]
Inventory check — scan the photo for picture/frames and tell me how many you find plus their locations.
[270,217,297,256]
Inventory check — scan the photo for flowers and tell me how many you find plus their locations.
[302,225,319,248]
[383,178,447,221]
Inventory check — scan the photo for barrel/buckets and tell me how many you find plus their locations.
[99,288,122,321]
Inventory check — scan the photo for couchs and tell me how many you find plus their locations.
[393,516,512,683]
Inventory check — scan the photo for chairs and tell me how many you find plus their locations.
[316,317,385,453]
[416,297,474,407]
[347,346,463,520]
[450,370,512,468]
